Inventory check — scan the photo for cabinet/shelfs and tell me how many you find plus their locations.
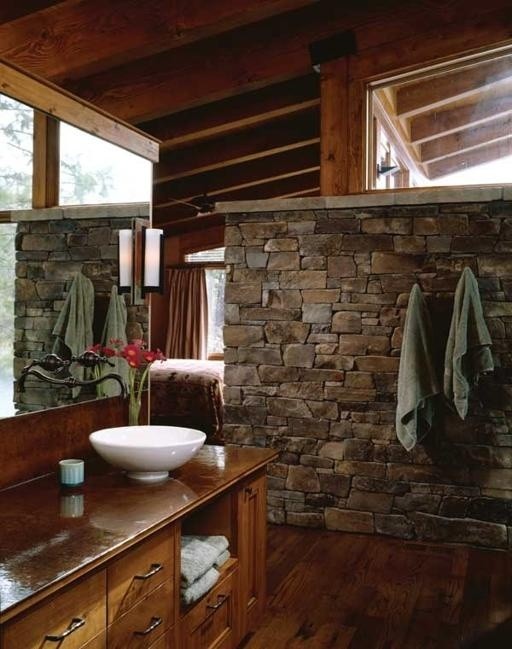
[103,518,178,648]
[181,459,267,635]
[0,566,108,647]
[179,555,247,648]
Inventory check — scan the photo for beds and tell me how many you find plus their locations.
[153,355,224,431]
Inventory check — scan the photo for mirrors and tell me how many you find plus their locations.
[0,57,165,426]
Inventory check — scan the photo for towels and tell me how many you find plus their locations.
[50,271,98,400]
[90,280,130,401]
[182,531,232,606]
[396,281,443,455]
[442,253,495,423]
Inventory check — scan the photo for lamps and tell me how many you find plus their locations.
[141,225,164,300]
[377,162,401,179]
[116,226,136,296]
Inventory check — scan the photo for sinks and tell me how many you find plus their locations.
[87,425,207,480]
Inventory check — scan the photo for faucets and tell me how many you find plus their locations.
[76,372,131,401]
[16,369,72,394]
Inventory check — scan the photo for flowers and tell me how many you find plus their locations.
[84,335,147,395]
[116,340,166,427]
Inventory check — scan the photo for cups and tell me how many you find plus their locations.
[56,457,85,488]
